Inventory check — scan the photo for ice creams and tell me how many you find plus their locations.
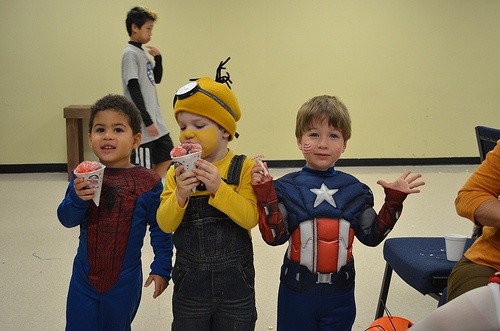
[170,142,202,193]
[73,160,106,206]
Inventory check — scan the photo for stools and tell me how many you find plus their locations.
[63,105,97,182]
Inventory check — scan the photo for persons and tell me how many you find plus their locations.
[250,95,425,331]
[156,76,258,331]
[57,93,174,331]
[121,6,174,178]
[403,139,500,331]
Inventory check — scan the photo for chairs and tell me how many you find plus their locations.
[374,126,500,321]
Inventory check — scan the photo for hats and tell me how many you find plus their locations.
[173,77,241,141]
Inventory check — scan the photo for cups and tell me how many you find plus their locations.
[171,151,202,193]
[74,165,106,207]
[444,233,467,261]
